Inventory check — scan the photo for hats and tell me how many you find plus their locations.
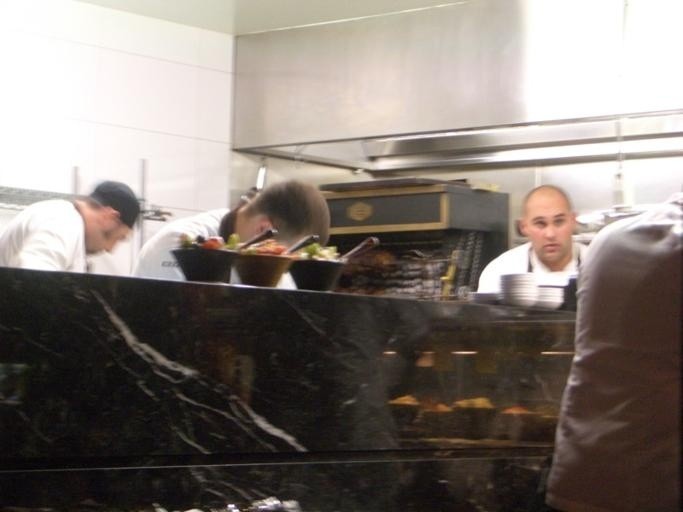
[88,180,141,230]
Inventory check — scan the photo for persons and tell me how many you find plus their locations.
[133,179,331,293]
[1,178,140,275]
[544,186,683,512]
[474,185,593,291]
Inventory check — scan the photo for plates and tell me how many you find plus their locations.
[501,274,562,313]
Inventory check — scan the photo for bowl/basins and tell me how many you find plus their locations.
[170,249,342,290]
